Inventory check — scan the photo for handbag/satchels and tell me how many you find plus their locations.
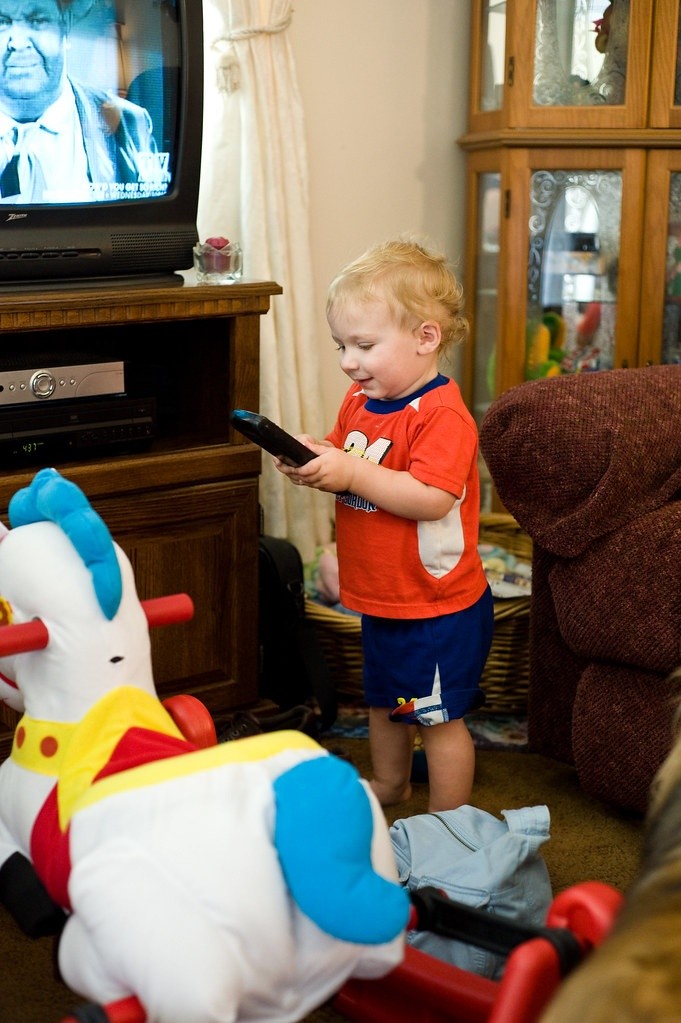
[389,805,551,981]
[258,533,338,734]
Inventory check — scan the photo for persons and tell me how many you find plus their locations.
[273,238,494,811]
[1,0,166,206]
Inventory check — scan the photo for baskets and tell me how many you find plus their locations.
[303,513,534,718]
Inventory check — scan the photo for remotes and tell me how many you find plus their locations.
[227,409,355,495]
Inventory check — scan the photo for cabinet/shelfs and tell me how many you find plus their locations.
[0,274,282,715]
[455,0,680,442]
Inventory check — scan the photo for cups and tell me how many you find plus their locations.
[192,241,243,286]
[589,70,626,105]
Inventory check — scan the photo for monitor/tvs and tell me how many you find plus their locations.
[0,0,203,294]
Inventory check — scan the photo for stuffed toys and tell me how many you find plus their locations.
[2,468,409,1023]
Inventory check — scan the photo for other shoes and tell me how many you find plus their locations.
[328,744,352,762]
[216,704,319,747]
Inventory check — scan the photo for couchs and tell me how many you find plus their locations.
[480,362,680,825]
